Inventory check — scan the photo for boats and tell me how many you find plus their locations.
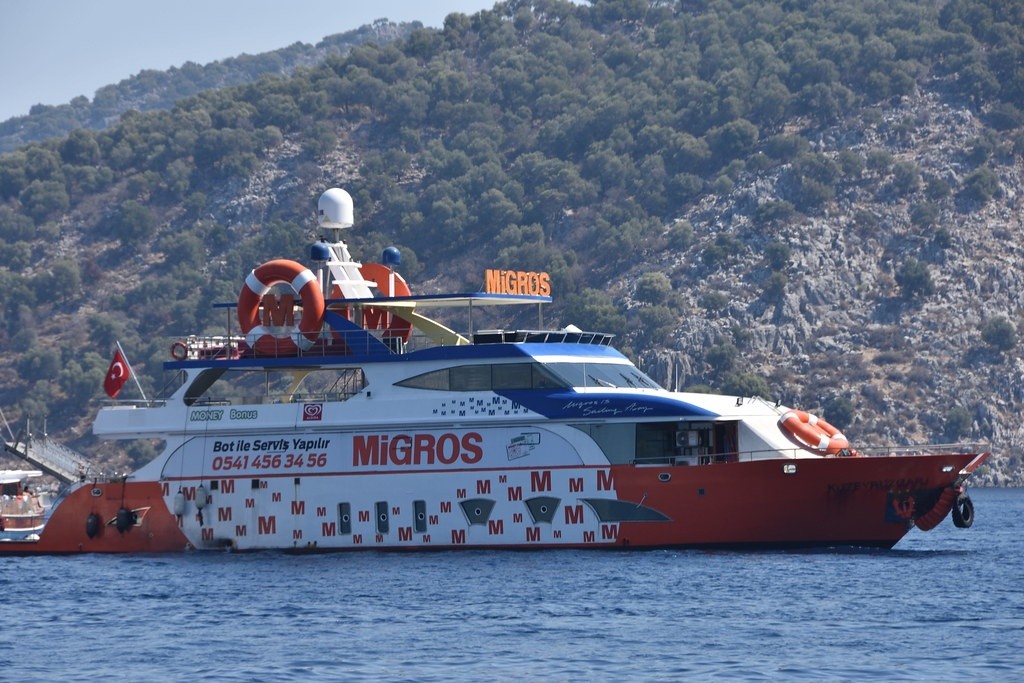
[1,188,995,557]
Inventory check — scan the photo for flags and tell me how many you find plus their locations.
[104,350,132,398]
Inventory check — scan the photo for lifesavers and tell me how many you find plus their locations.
[235,258,319,354]
[782,409,849,453]
[952,490,975,527]
[171,341,187,361]
